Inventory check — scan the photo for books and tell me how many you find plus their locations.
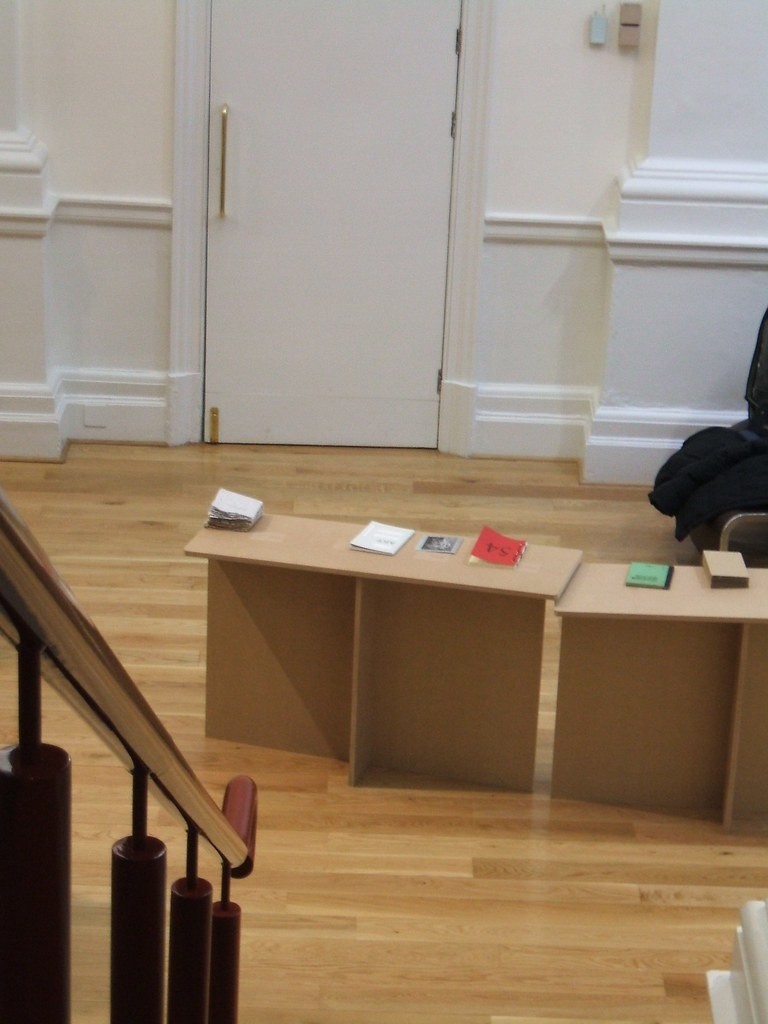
[204,488,263,532]
[350,521,415,556]
[626,561,675,590]
[468,526,528,569]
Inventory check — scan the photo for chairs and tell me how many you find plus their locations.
[647,306,768,557]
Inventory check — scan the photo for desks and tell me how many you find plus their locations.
[552,559,767,838]
[184,512,584,794]
